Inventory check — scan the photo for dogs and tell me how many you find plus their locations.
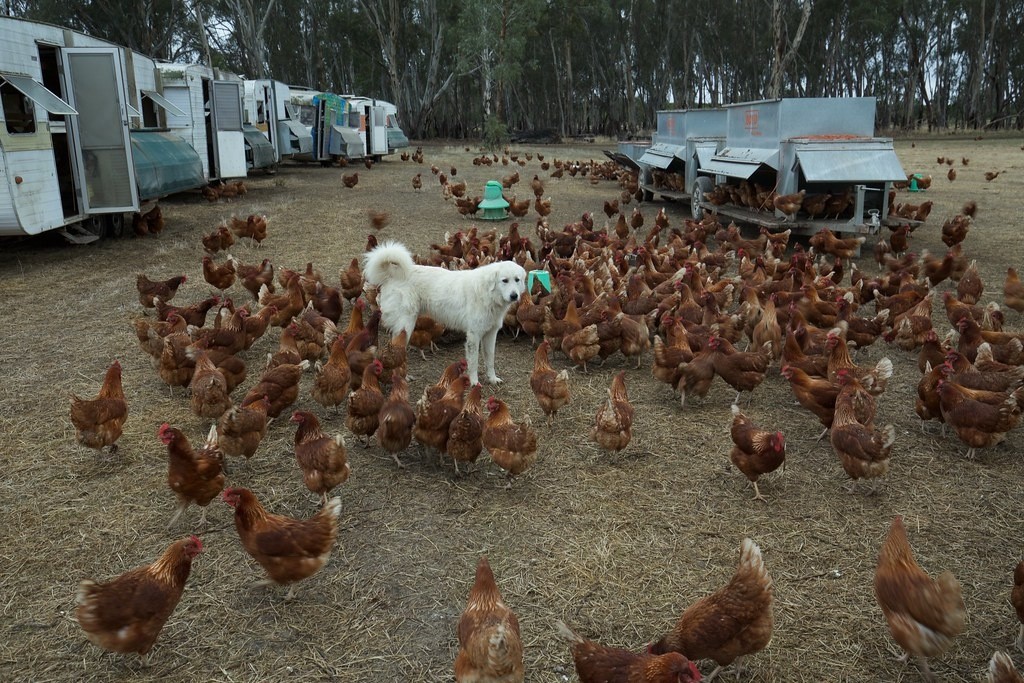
[356,237,529,390]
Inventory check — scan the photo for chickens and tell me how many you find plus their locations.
[64,141,1021,682]
